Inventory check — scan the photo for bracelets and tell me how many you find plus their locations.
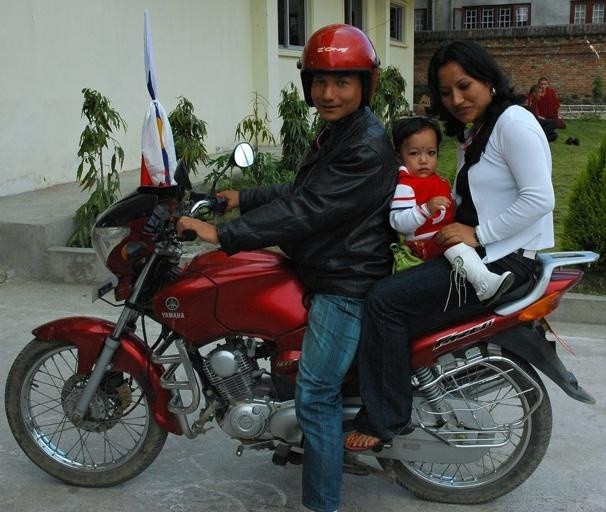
[472,225,482,248]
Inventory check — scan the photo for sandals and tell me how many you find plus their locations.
[342,429,383,452]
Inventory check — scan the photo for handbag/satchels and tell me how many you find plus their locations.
[388,242,425,275]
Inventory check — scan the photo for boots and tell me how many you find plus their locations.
[444,241,517,310]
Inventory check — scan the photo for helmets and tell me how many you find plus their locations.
[297,24,379,106]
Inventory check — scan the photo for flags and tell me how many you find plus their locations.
[137,7,179,188]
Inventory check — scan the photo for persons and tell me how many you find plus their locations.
[387,107,518,311]
[169,21,401,512]
[538,77,567,130]
[516,94,531,110]
[527,86,558,142]
[339,36,559,457]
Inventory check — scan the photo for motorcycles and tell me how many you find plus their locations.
[3,142,601,504]
